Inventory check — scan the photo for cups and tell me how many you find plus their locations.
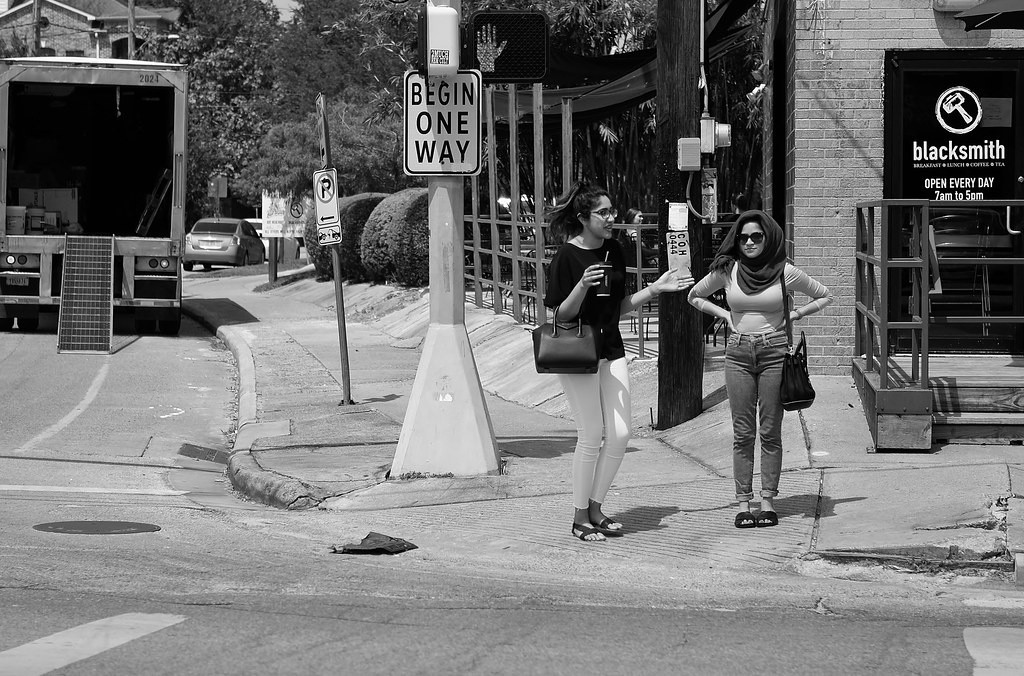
[595,261,611,296]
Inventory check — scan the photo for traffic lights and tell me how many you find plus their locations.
[467,9,550,81]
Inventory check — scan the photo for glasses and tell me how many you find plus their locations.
[591,208,618,217]
[737,232,765,245]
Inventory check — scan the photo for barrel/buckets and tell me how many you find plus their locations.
[6,206,26,235]
[26,205,45,234]
[45,210,63,235]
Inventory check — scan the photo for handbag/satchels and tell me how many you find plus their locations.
[780,331,816,411]
[532,306,598,375]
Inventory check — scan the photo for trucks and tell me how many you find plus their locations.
[1,55,188,338]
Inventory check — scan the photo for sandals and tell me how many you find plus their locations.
[757,511,777,527]
[735,511,756,528]
[593,518,618,533]
[572,522,606,542]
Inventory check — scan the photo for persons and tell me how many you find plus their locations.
[687,210,834,528]
[543,182,680,541]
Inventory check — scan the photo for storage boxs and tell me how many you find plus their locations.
[19,187,78,222]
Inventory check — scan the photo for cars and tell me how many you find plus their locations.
[182,218,266,271]
[243,218,300,263]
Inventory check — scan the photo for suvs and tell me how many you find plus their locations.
[910,208,1011,279]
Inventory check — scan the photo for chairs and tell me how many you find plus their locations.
[506,230,729,351]
